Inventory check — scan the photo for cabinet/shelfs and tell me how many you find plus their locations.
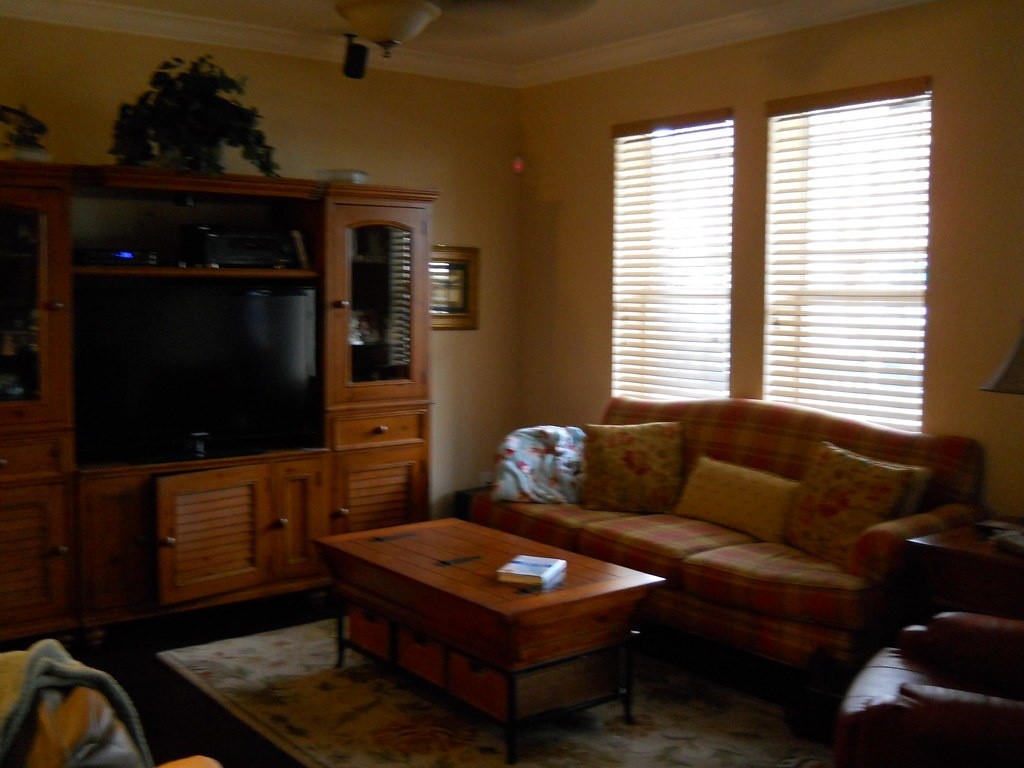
[0,159,436,649]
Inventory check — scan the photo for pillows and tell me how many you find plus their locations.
[787,442,936,562]
[584,423,684,515]
[677,455,802,545]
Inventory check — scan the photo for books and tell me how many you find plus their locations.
[496,555,567,585]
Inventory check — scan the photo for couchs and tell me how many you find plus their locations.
[469,397,986,746]
[833,612,1024,768]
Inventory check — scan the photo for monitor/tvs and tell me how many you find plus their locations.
[73,273,326,465]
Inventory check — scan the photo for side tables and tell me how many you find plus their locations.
[905,516,1024,626]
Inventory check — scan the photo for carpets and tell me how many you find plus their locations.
[157,618,833,768]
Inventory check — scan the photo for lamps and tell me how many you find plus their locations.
[334,0,441,58]
[978,324,1024,556]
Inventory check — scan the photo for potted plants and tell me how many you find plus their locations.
[108,53,281,180]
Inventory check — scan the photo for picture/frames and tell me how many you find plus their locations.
[430,245,480,330]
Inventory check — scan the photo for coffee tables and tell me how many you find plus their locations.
[314,519,667,766]
